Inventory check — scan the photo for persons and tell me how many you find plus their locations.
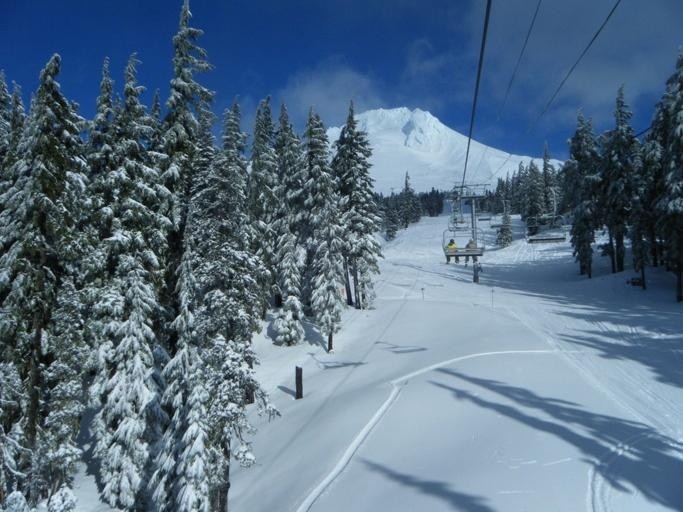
[463,238,478,269]
[453,212,458,226]
[446,238,459,265]
